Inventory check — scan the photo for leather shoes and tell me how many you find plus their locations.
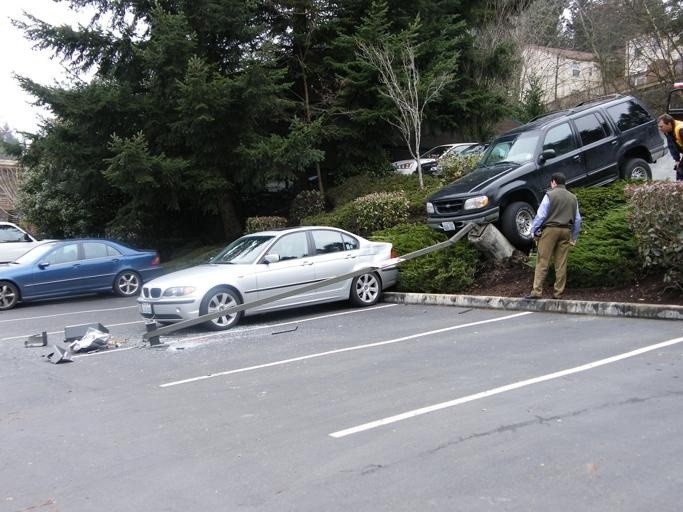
[525,294,542,299]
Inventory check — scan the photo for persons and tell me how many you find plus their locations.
[658,115,683,180]
[525,172,581,299]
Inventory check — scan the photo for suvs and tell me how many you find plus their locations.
[426,92,669,248]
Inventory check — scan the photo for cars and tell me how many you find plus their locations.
[0,222,61,266]
[421,142,489,183]
[0,240,165,310]
[136,226,400,331]
[390,142,479,178]
